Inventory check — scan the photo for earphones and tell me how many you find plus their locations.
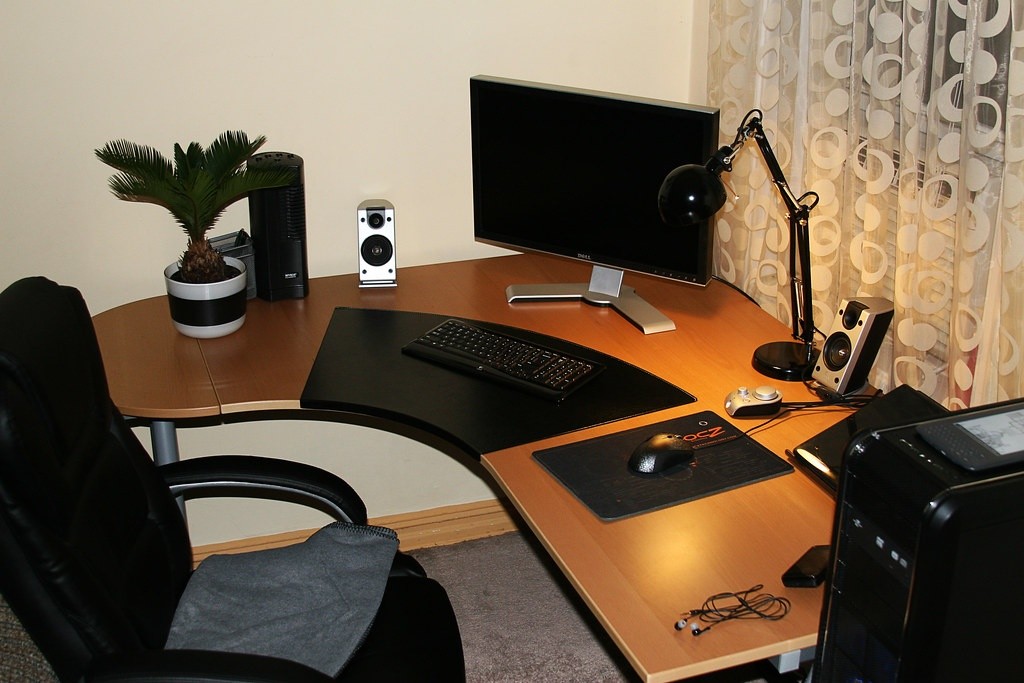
[674,618,688,631]
[691,623,711,635]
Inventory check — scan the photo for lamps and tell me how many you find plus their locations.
[657,107,822,382]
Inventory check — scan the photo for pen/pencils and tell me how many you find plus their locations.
[235,228,244,246]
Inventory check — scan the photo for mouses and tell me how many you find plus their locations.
[628,432,694,475]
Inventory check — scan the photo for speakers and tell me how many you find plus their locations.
[812,297,894,401]
[357,199,398,289]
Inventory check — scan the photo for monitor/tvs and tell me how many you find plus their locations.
[470,74,719,335]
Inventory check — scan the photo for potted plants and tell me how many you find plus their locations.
[94,130,297,340]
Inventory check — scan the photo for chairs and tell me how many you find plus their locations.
[0,275,469,683]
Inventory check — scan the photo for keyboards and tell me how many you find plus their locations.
[402,318,608,403]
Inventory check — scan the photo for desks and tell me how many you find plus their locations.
[92,253,859,683]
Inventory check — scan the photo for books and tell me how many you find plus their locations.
[792,383,949,495]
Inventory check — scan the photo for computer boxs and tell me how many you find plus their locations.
[813,397,1023,683]
